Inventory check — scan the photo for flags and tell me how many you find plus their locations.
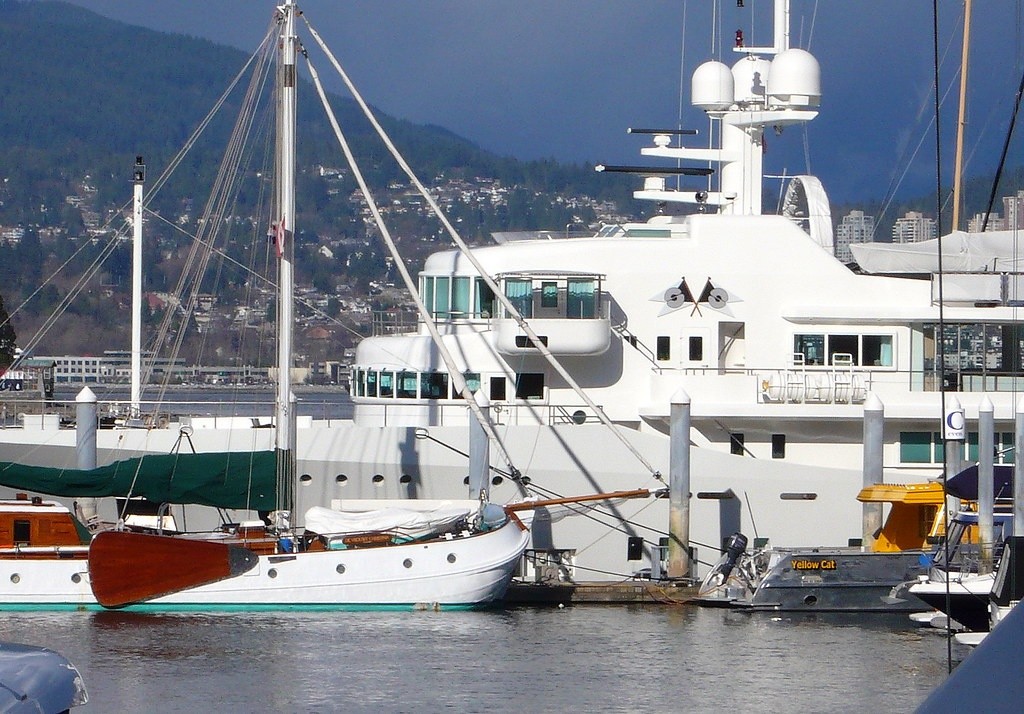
[275,215,285,257]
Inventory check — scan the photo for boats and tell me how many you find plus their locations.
[0,1,1024,653]
